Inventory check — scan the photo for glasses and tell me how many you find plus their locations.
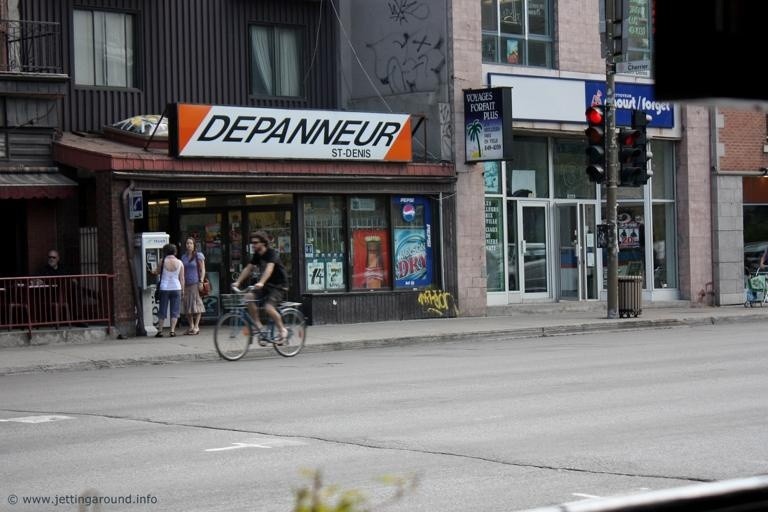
[250,242,264,244]
[48,256,56,259]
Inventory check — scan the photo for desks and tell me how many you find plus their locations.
[13,282,59,328]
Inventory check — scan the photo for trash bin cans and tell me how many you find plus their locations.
[618,276,643,319]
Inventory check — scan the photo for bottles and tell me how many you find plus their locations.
[363,237,387,290]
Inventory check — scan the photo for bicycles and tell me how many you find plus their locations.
[214,286,306,361]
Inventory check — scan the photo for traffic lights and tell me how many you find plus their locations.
[585,106,653,187]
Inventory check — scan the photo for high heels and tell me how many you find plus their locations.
[184,329,200,335]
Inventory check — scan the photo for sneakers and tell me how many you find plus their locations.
[279,328,288,338]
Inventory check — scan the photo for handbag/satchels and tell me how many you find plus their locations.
[155,282,161,304]
[199,277,210,296]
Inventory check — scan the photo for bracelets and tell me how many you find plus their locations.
[199,279,205,283]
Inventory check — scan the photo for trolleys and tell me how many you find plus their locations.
[744,266,768,308]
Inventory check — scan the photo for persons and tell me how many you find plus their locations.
[30,248,82,328]
[230,231,289,340]
[179,237,206,336]
[155,244,184,339]
[759,246,768,270]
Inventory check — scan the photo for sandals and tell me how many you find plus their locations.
[155,332,163,337]
[170,332,175,337]
